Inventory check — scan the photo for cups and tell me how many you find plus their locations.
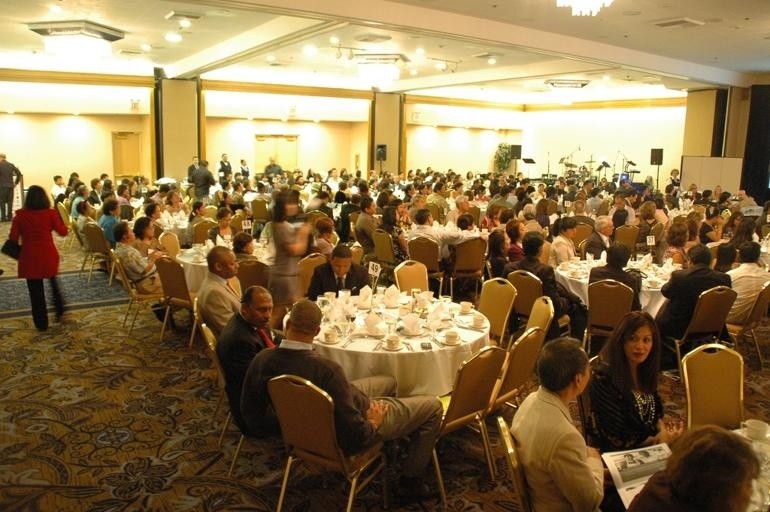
[738,418,769,439]
[558,255,683,289]
[401,225,489,239]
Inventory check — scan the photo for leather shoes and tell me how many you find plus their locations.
[395,483,432,504]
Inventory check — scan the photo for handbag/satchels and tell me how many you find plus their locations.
[1,239,20,259]
[625,268,648,291]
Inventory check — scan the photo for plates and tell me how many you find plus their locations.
[177,236,274,265]
[312,286,489,352]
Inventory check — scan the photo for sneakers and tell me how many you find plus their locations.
[151,304,166,322]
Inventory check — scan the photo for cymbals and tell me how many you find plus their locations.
[558,156,565,164]
[565,163,577,167]
[584,161,595,163]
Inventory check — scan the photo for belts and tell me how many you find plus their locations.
[134,271,156,282]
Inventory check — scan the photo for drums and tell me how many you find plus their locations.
[566,171,597,187]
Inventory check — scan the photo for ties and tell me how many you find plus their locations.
[229,283,240,298]
[256,326,275,348]
[335,276,344,289]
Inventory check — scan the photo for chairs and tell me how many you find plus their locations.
[37,167,770,511]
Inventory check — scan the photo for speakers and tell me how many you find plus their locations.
[510,145,522,159]
[651,148,663,165]
[376,144,387,161]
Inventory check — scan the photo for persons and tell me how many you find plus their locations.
[7,185,67,333]
[0,152,22,221]
[55,141,769,510]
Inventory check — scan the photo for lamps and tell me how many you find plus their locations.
[546,77,592,109]
[553,1,618,17]
[346,52,413,87]
[28,21,125,61]
